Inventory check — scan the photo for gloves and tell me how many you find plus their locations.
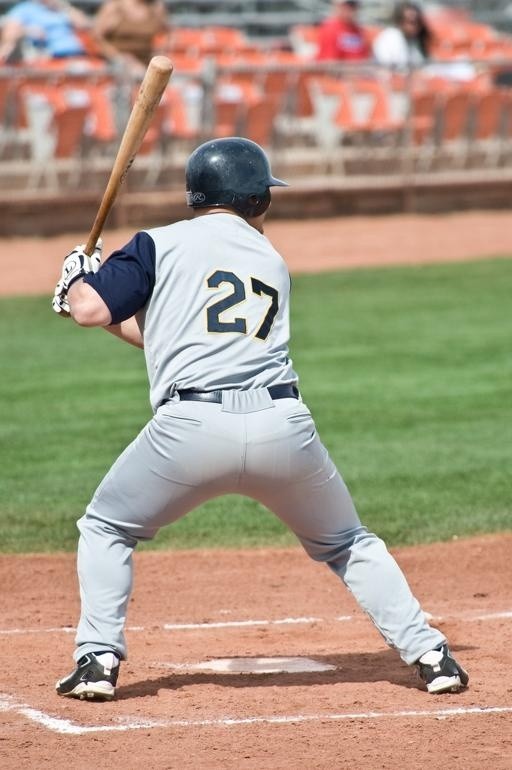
[52,239,103,313]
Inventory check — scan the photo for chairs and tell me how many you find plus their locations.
[0,11,512,162]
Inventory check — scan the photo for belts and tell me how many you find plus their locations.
[178,384,299,405]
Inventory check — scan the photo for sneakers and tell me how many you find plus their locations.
[416,645,469,694]
[55,650,120,702]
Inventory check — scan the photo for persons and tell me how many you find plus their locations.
[0,0,166,60]
[54,137,467,700]
[312,0,432,60]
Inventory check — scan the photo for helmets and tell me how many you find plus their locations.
[185,137,291,218]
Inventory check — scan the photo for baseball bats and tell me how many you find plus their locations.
[84,54,173,255]
